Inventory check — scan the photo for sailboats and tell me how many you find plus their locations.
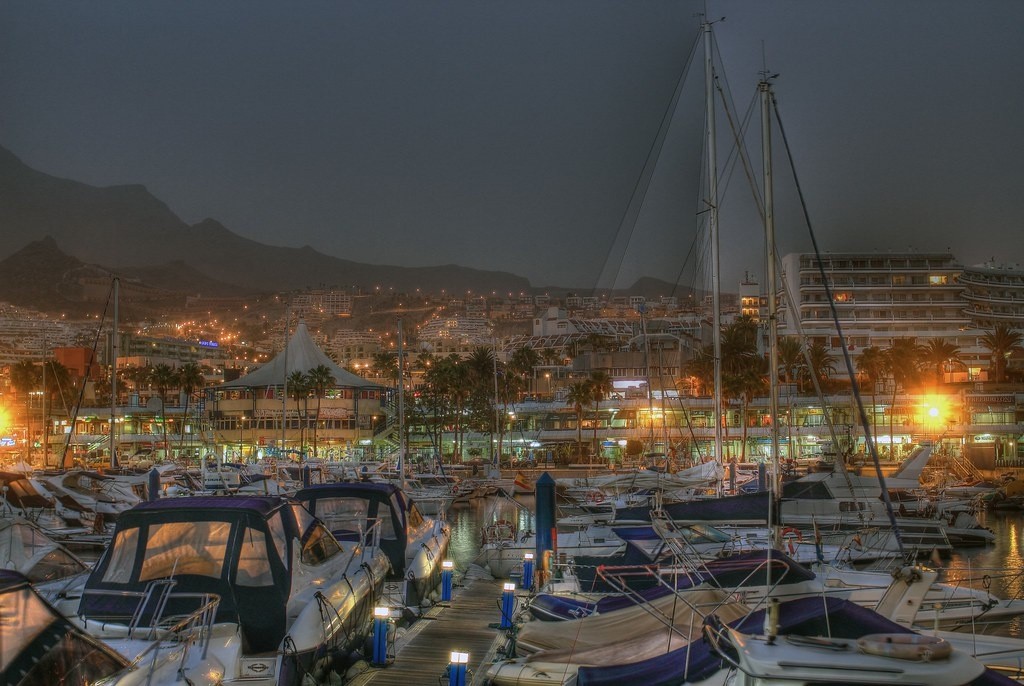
[0,9,1024,685]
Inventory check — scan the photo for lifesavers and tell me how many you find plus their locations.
[453,486,458,490]
[782,527,802,543]
[543,550,556,576]
[481,527,486,545]
[856,631,953,660]
[793,461,798,468]
[593,492,604,502]
[493,520,514,539]
